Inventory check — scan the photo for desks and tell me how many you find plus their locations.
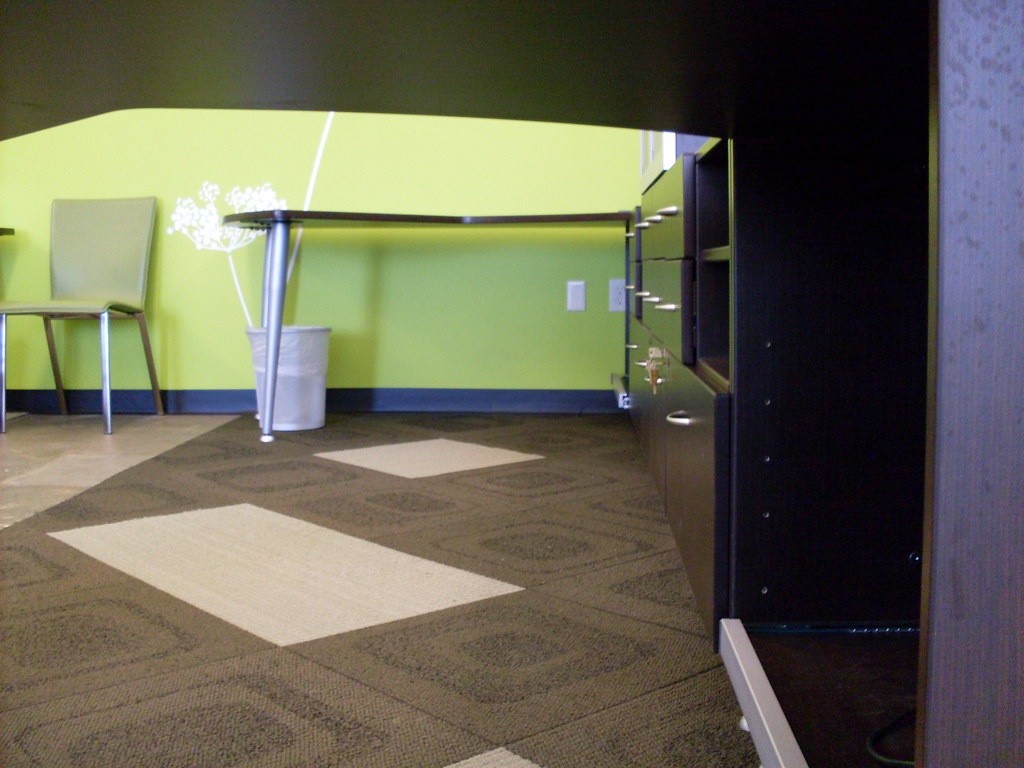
[222,208,635,441]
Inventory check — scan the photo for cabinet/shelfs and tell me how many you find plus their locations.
[628,139,928,653]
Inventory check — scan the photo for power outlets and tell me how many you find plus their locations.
[610,279,627,312]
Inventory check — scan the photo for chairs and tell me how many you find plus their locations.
[1,196,165,434]
